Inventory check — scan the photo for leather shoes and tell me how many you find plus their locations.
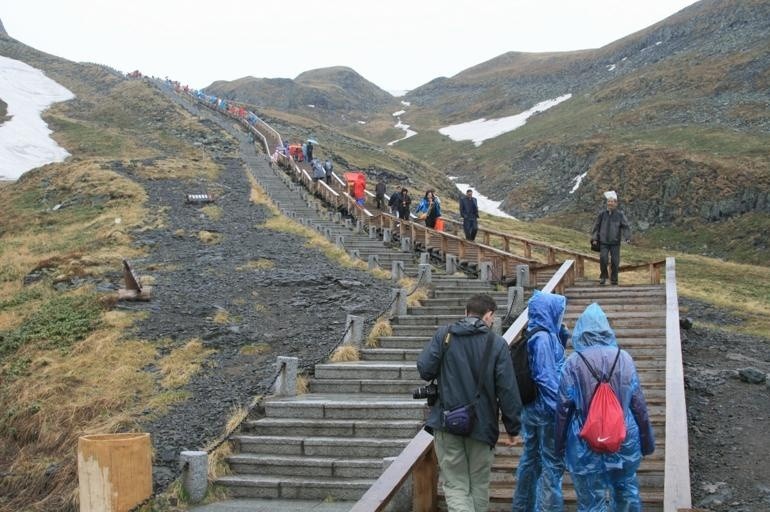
[600,278,618,284]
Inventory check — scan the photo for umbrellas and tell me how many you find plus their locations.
[303,139,319,146]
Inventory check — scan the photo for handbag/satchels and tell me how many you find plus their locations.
[419,213,426,222]
[591,239,600,252]
[442,399,474,436]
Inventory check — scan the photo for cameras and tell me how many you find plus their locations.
[413,384,437,406]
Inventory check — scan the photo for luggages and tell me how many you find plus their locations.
[433,216,445,231]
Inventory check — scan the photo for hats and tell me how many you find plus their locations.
[604,191,617,201]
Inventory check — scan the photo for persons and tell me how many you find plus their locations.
[415,190,441,229]
[281,141,314,162]
[374,178,388,211]
[415,294,525,511]
[325,160,333,185]
[551,301,656,511]
[353,174,367,206]
[398,189,412,220]
[430,188,440,204]
[388,185,402,216]
[311,157,325,182]
[589,189,632,286]
[169,79,259,128]
[510,287,571,511]
[458,189,481,241]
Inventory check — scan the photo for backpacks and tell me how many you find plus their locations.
[510,325,542,405]
[579,349,626,453]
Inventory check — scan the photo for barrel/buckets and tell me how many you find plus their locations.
[76,432,152,510]
